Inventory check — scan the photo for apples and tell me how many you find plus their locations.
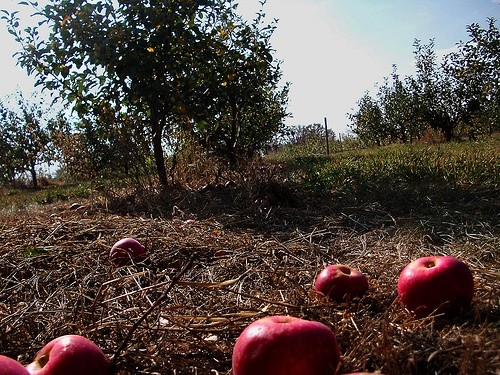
[232,316,339,375]
[399,256,473,319]
[110,237,146,262]
[0,333,111,375]
[315,263,369,300]
